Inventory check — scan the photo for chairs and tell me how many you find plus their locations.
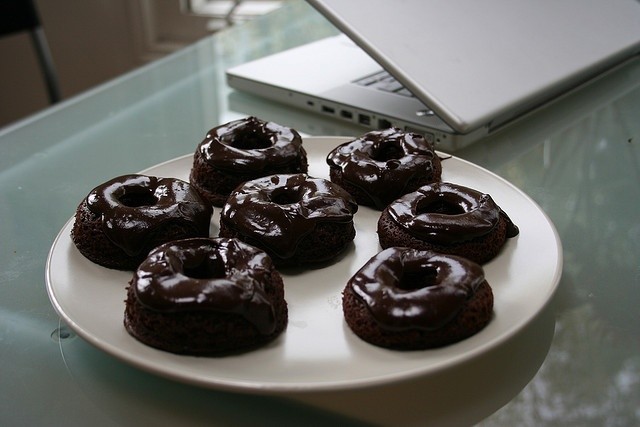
[0,0,64,112]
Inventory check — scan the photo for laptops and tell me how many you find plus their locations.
[226,0,640,153]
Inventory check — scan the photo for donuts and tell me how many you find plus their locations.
[189,112,310,207]
[122,236,289,358]
[71,172,215,271]
[217,172,358,272]
[340,246,494,350]
[324,124,442,210]
[379,180,523,263]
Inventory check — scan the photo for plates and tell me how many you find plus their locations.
[44,136,564,395]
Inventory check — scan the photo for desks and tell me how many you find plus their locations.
[0,0,640,427]
